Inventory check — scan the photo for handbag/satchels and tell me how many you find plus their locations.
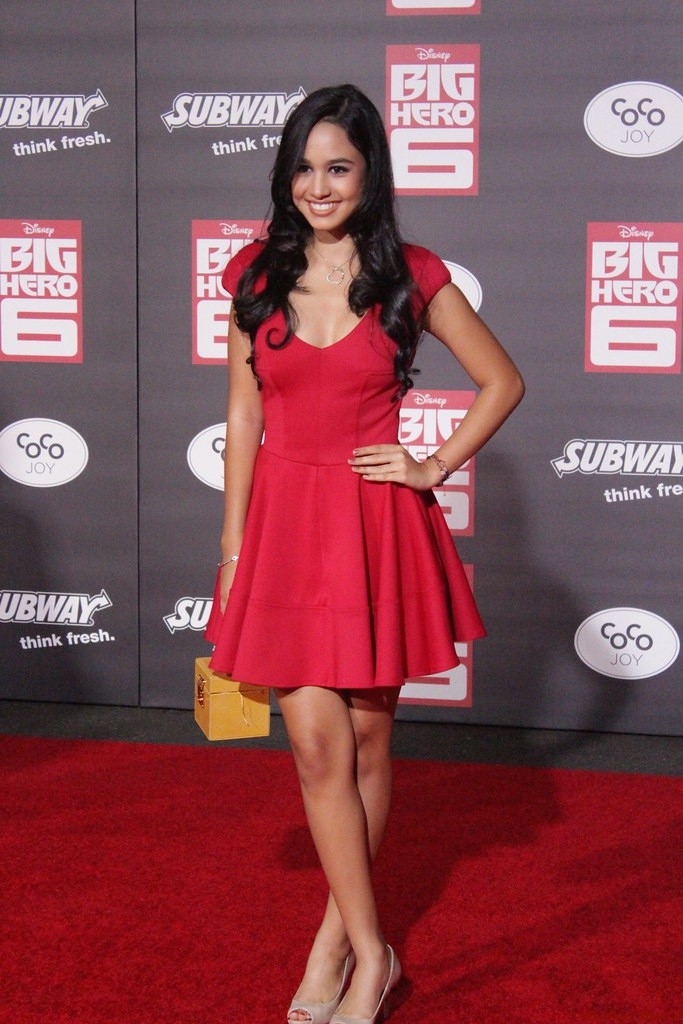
[193,656,272,742]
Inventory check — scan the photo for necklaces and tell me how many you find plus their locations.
[308,235,358,284]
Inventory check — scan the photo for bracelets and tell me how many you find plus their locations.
[217,555,239,568]
[427,453,451,487]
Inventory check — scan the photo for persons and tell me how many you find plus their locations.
[203,83,527,1024]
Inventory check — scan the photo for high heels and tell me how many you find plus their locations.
[287,949,358,1024]
[328,943,402,1024]
